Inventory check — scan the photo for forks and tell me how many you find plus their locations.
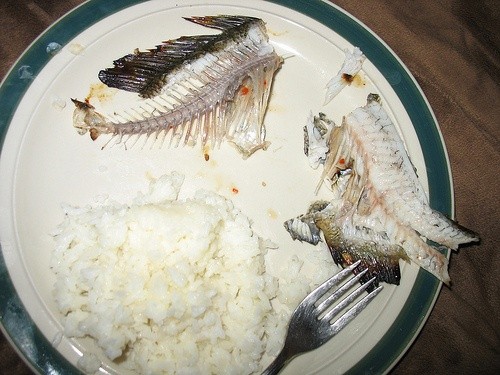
[260,260,384,375]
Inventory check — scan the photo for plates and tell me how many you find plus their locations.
[0,0,455,374]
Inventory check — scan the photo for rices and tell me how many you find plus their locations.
[44,169,313,375]
